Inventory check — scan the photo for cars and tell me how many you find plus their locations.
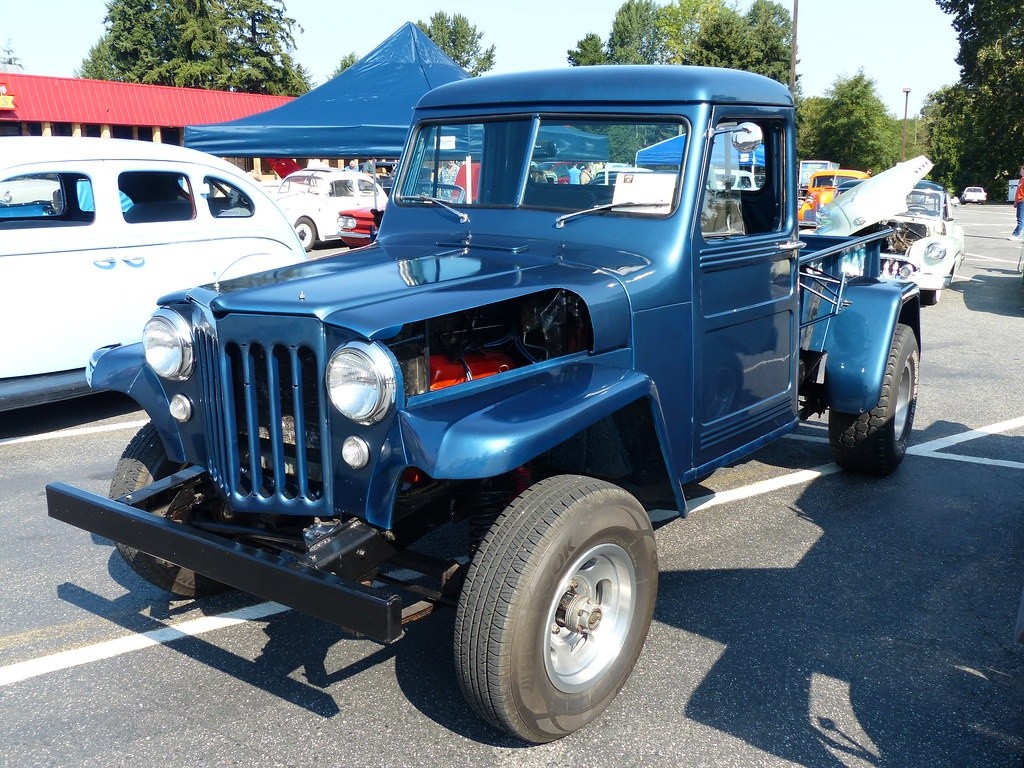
[799,169,873,233]
[819,180,965,305]
[272,166,389,250]
[713,169,760,191]
[0,135,309,415]
[961,187,987,204]
[358,162,435,199]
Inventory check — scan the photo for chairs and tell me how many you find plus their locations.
[701,199,745,235]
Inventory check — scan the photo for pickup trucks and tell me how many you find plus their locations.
[46,64,922,746]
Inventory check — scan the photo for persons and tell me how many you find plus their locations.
[345,156,592,205]
[1007,165,1024,241]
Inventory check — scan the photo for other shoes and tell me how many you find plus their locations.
[1007,235,1018,240]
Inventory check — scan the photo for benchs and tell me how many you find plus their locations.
[124,200,252,222]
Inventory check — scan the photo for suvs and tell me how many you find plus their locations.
[538,161,586,186]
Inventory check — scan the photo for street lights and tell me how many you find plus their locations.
[902,88,911,162]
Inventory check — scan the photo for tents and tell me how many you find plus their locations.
[634,128,766,171]
[183,20,609,211]
